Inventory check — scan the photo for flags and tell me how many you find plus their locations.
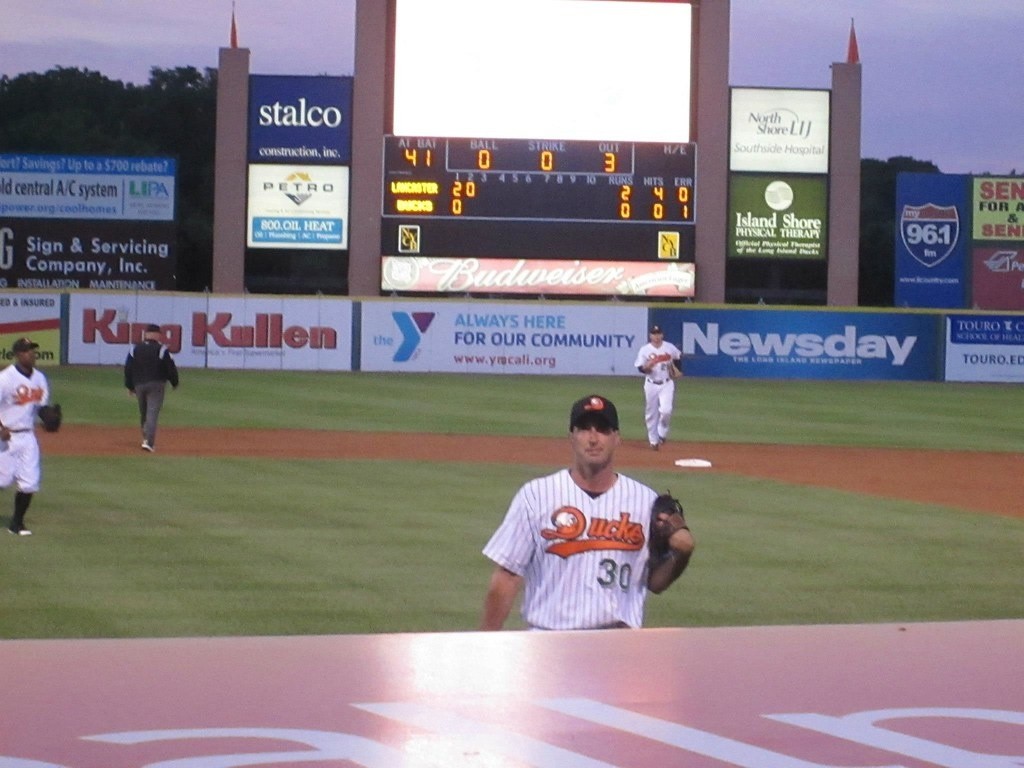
[231,17,237,48]
[848,26,859,63]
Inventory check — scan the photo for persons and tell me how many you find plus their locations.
[123,324,179,452]
[0,338,62,535]
[634,326,682,450]
[483,396,695,630]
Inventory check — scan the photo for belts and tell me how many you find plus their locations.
[648,378,669,384]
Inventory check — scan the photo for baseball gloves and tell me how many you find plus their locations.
[38,404,62,433]
[648,491,694,561]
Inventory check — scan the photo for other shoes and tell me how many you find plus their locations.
[7,525,32,535]
[650,444,659,450]
[659,436,666,443]
[141,439,154,452]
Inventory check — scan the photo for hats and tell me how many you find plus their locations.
[570,395,618,431]
[650,325,661,334]
[148,324,160,332]
[12,337,39,352]
